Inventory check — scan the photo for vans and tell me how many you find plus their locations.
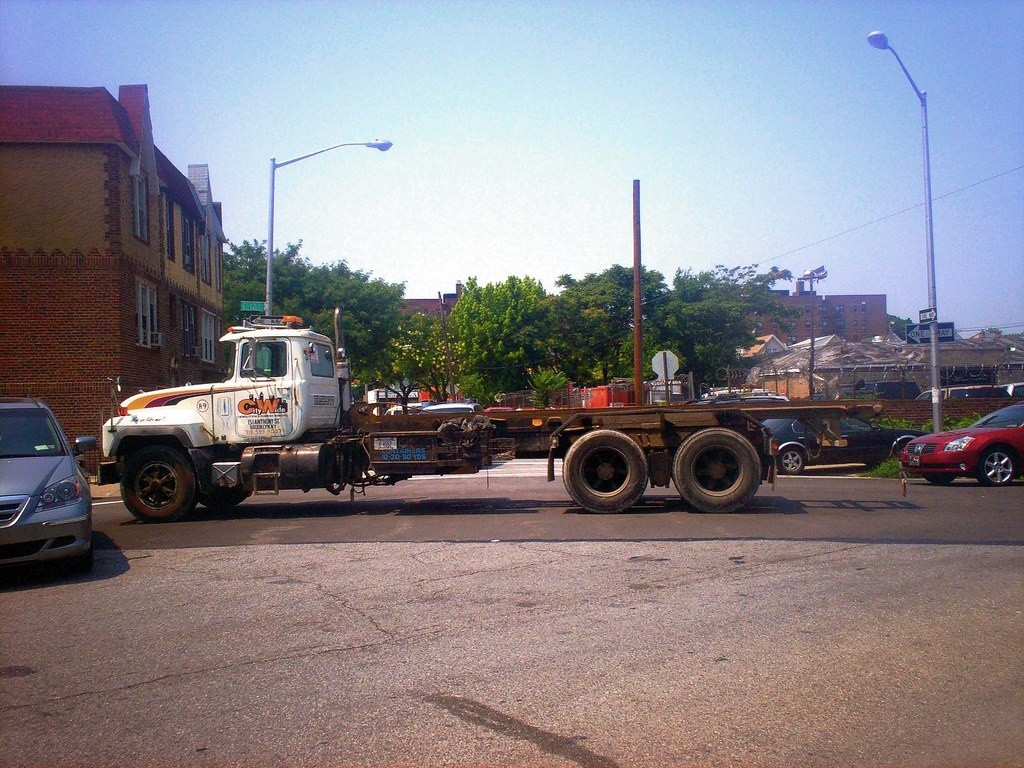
[419,402,484,414]
[381,403,426,412]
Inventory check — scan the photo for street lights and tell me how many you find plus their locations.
[867,30,944,433]
[264,140,396,316]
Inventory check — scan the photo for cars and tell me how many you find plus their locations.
[898,399,1024,486]
[1,399,99,573]
[760,415,931,476]
[481,407,515,411]
[682,385,790,404]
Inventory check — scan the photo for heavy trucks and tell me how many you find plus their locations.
[99,314,882,523]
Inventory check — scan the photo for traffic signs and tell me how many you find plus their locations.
[918,306,938,323]
[905,322,955,344]
